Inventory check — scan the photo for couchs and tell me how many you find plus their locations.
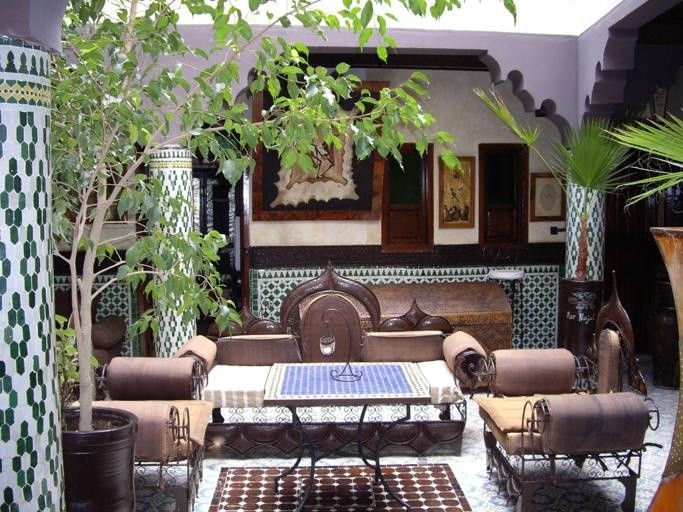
[172,256,487,422]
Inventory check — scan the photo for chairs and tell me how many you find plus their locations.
[470,269,663,511]
[61,356,214,491]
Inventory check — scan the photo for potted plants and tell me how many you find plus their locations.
[50,0,518,512]
[598,108,682,512]
[473,82,635,393]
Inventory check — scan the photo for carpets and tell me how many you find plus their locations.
[209,463,472,512]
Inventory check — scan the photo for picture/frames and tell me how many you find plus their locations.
[530,172,567,221]
[439,156,475,228]
[252,80,391,220]
